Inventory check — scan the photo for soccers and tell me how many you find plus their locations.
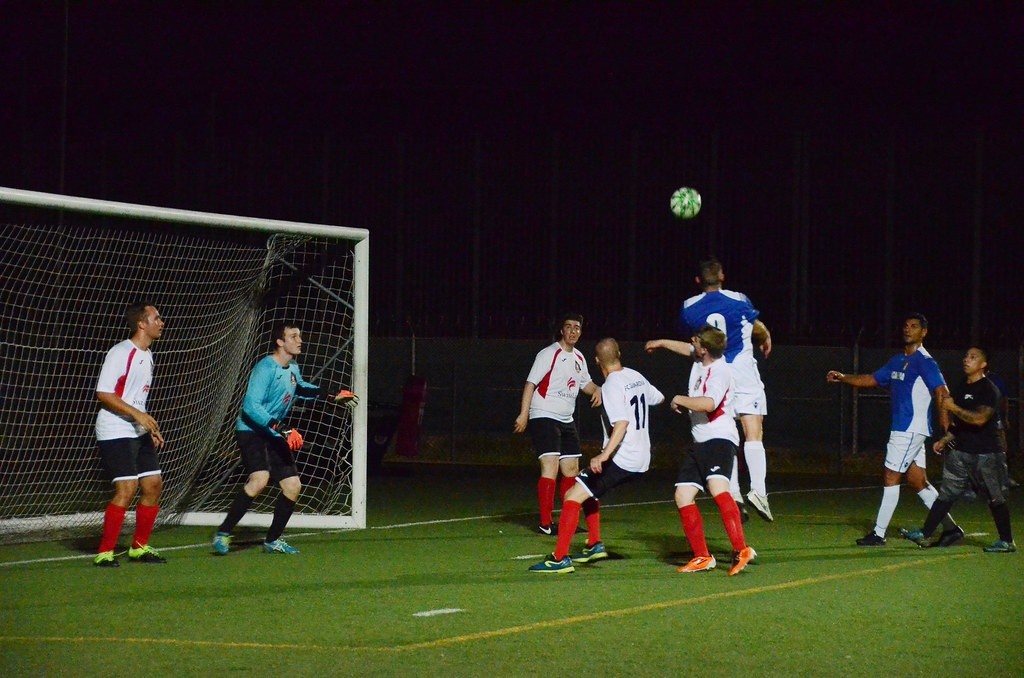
[670,188,702,218]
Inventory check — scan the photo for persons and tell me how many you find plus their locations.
[92,301,167,567]
[677,257,773,524]
[211,321,361,555]
[644,324,757,576]
[896,347,1017,553]
[512,312,601,535]
[528,338,666,573]
[826,312,965,548]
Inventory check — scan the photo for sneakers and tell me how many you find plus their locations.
[931,525,964,547]
[732,492,749,524]
[856,531,887,545]
[677,554,717,572]
[572,541,608,563]
[748,488,774,522]
[983,539,1017,552]
[538,521,558,535]
[263,538,300,554]
[93,549,114,566]
[728,546,757,576]
[529,552,575,573]
[575,526,588,533]
[211,531,231,555]
[128,544,168,563]
[900,528,931,547]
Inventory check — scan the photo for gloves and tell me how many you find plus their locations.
[326,389,359,409]
[279,425,304,452]
